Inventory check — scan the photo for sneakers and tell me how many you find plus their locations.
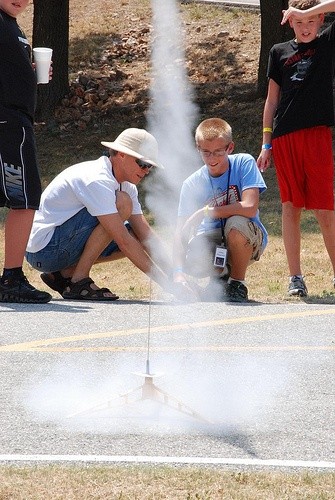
[288,275,307,295]
[0,276,52,303]
[205,266,229,300]
[219,280,248,302]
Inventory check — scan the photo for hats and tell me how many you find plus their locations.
[101,128,165,169]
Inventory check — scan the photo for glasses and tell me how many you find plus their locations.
[199,141,232,157]
[136,159,152,168]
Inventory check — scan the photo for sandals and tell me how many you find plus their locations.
[63,278,119,300]
[40,271,70,296]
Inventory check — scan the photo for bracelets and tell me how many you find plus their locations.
[263,144,272,148]
[204,205,209,217]
[263,128,272,132]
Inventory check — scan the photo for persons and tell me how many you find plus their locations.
[257,0,335,296]
[25,128,198,302]
[0,0,52,304]
[170,118,268,302]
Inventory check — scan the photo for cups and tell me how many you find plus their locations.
[32,46,53,84]
